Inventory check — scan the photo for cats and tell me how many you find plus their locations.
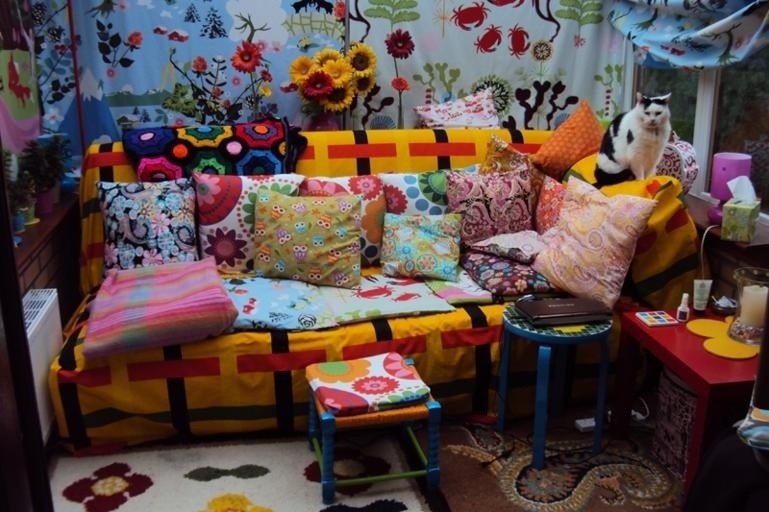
[593,91,673,189]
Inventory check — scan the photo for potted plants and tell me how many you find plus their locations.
[3,135,75,247]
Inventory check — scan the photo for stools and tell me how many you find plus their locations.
[495,295,622,471]
[305,352,443,505]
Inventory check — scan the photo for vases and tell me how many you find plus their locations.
[310,110,341,130]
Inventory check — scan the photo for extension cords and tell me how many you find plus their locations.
[574,409,642,432]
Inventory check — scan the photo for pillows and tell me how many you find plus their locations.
[413,86,499,126]
[531,100,604,181]
[378,164,480,215]
[446,164,532,243]
[530,174,658,312]
[534,175,565,235]
[94,177,198,278]
[191,171,305,275]
[253,188,362,292]
[380,211,463,282]
[299,174,386,268]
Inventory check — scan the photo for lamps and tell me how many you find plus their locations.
[707,152,752,226]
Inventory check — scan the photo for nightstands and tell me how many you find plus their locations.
[623,305,763,497]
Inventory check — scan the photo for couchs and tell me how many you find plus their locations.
[49,121,711,458]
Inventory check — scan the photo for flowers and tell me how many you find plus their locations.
[289,43,373,111]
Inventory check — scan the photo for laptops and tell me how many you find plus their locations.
[515,296,613,327]
